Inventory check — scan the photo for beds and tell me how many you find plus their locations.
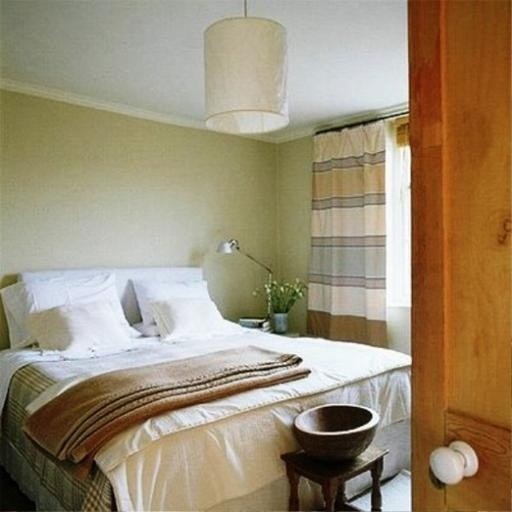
[0,267,413,512]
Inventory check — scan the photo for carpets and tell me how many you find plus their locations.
[345,469,412,512]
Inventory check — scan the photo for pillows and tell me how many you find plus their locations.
[1,273,225,359]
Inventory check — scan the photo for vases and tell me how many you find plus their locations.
[294,402,380,463]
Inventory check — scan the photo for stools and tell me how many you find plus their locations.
[281,444,391,512]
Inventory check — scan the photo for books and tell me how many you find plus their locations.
[239,318,274,334]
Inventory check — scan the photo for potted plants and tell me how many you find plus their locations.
[253,277,309,333]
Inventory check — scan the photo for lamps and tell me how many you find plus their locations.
[216,239,274,329]
[202,1,292,137]
